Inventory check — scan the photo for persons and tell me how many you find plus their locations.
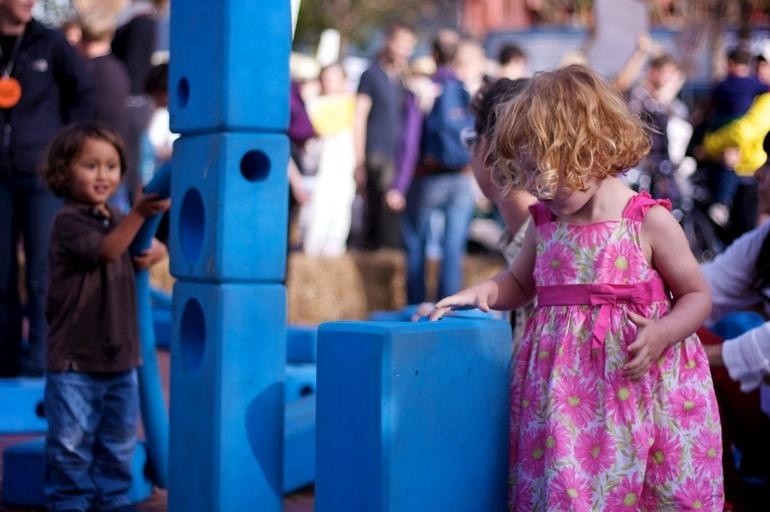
[0,0,180,385]
[700,130,770,393]
[42,119,171,511]
[288,24,652,304]
[624,45,770,259]
[415,63,725,512]
